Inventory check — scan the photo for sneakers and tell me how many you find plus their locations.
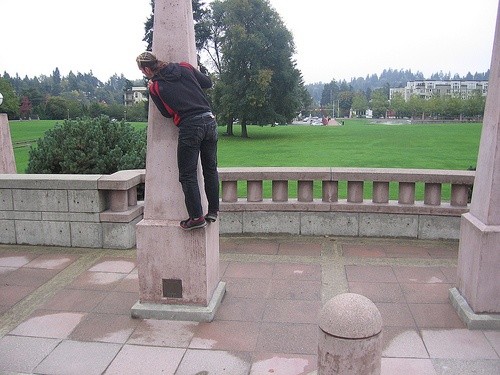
[180,216,206,231]
[205,210,218,222]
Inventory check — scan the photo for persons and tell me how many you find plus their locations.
[135,52,220,232]
[310,115,345,127]
